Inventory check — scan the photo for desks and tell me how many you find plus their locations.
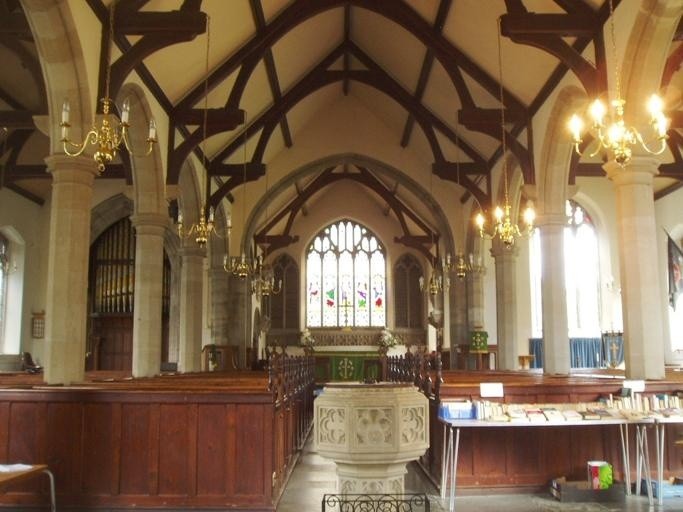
[439,401,683,511]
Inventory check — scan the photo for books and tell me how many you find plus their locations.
[474,400,566,422]
[577,411,601,419]
[562,409,583,420]
[597,393,681,417]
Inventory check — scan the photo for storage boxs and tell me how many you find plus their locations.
[550,476,625,503]
[638,475,682,498]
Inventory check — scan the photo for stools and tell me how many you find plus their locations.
[0,463,61,512]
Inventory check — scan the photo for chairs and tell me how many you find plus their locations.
[20,351,42,372]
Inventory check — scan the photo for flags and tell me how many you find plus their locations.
[668,236,683,311]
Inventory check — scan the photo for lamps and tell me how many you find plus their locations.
[442,111,486,282]
[0,241,19,279]
[252,170,283,302]
[58,0,161,176]
[223,113,258,282]
[177,16,234,251]
[567,1,672,169]
[476,20,539,251]
[419,166,452,297]
